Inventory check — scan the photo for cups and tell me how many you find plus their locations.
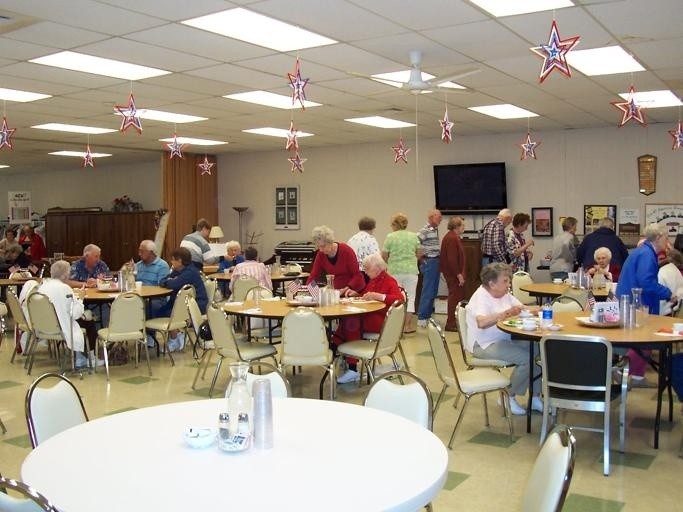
[95,272,117,292]
[639,304,650,321]
[223,269,229,277]
[245,287,317,306]
[672,322,683,334]
[135,281,141,289]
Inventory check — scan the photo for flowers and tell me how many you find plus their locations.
[111,195,143,213]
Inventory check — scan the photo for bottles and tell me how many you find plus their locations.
[631,289,641,327]
[117,271,124,292]
[235,412,252,451]
[264,255,288,278]
[252,377,274,448]
[318,274,340,307]
[590,301,619,323]
[216,412,234,451]
[542,299,552,328]
[551,263,611,291]
[124,260,134,294]
[288,256,314,261]
[224,362,254,443]
[619,294,629,327]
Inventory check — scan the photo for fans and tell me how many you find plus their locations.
[349,51,482,95]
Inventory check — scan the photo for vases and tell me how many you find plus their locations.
[243,244,261,262]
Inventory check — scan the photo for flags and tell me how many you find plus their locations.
[288,278,321,298]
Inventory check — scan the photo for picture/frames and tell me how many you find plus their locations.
[637,154,657,195]
[584,205,617,235]
[644,202,683,237]
[531,206,554,237]
[273,184,299,230]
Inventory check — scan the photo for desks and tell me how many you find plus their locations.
[413,239,482,314]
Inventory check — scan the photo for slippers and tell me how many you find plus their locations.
[404,330,416,333]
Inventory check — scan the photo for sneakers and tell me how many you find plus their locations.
[148,331,189,353]
[87,358,105,368]
[629,377,657,388]
[445,326,457,332]
[416,319,427,327]
[530,398,551,414]
[498,391,526,416]
[612,365,622,381]
[337,370,361,383]
[76,356,88,367]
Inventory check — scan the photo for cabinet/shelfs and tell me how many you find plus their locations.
[45,211,166,271]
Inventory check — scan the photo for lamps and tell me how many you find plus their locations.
[209,226,224,243]
[232,207,249,249]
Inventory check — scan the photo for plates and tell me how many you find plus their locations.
[185,428,215,449]
[502,309,542,332]
[575,316,622,328]
[547,324,560,331]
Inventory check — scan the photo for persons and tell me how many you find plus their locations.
[550,217,682,386]
[465,210,553,415]
[2,226,112,366]
[307,225,404,383]
[347,209,467,331]
[134,222,235,353]
[218,241,274,328]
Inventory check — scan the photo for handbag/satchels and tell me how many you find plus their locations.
[200,318,213,342]
[99,341,129,366]
